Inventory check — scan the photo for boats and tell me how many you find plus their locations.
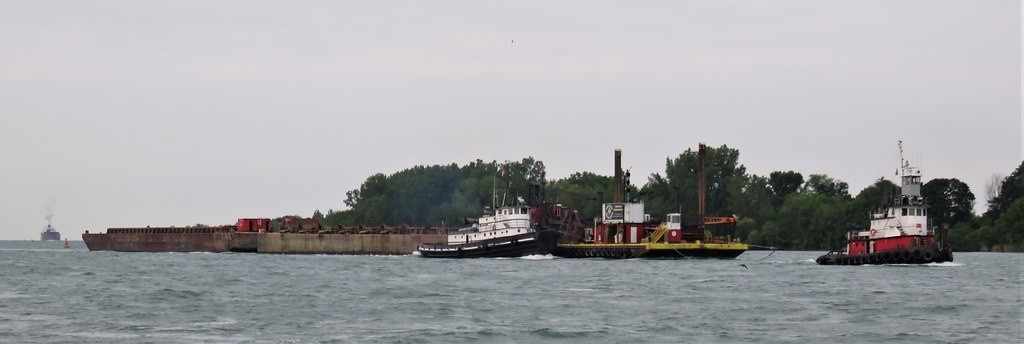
[40,224,62,241]
[560,141,749,260]
[816,140,956,268]
[418,176,590,260]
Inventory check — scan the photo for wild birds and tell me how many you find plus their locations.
[737,263,748,269]
